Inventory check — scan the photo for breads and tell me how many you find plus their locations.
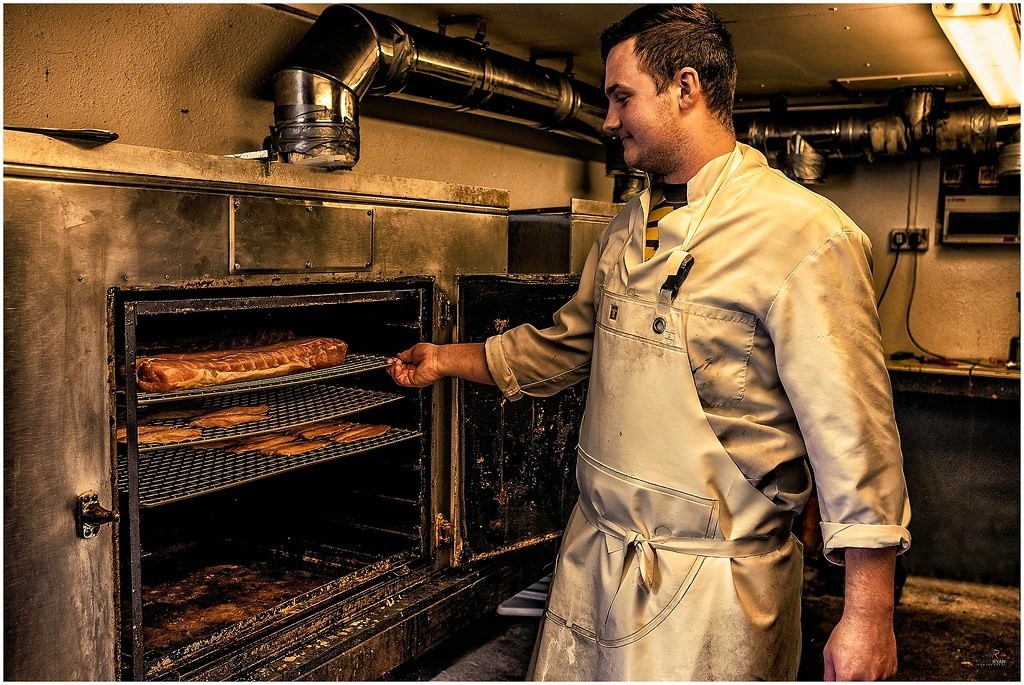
[115,330,348,392]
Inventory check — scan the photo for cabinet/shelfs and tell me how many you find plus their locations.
[113,353,424,503]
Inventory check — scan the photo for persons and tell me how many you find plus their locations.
[385,3,913,681]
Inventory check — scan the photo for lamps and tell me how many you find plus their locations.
[931,2,1021,109]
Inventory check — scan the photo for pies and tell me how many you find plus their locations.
[112,403,390,455]
